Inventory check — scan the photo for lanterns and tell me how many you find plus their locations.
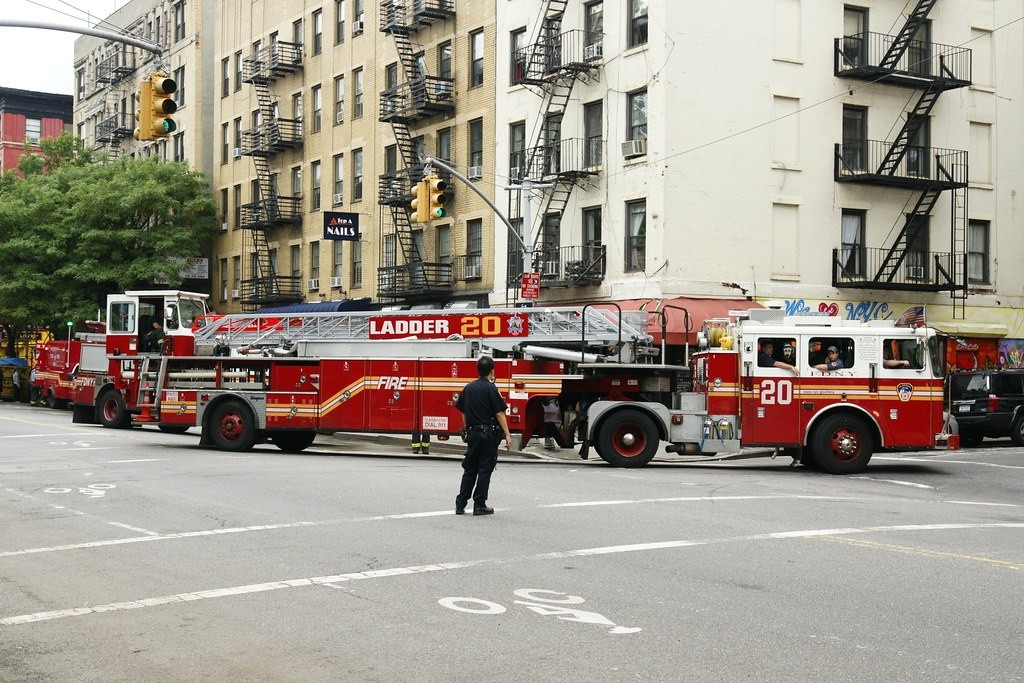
[0,358,28,367]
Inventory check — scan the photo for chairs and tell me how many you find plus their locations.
[138,315,153,336]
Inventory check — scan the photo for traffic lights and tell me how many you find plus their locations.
[411,183,430,224]
[149,73,178,138]
[430,179,447,220]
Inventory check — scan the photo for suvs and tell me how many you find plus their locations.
[944,365,1024,447]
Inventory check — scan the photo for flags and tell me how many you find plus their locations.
[895,307,924,325]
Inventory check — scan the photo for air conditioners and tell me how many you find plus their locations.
[468,166,482,179]
[231,49,453,299]
[463,266,479,278]
[510,167,520,181]
[543,262,560,276]
[585,43,602,59]
[353,21,363,32]
[906,267,924,279]
[622,139,645,156]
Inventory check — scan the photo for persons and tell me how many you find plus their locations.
[883,342,910,369]
[12,368,23,403]
[455,356,511,515]
[30,367,48,405]
[0,367,4,402]
[758,339,800,376]
[412,433,430,454]
[813,346,843,371]
[144,322,165,352]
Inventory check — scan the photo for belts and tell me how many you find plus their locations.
[470,424,501,433]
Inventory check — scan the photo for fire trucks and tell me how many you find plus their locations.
[34,290,961,475]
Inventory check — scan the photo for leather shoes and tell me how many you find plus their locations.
[473,501,494,514]
[456,506,465,514]
